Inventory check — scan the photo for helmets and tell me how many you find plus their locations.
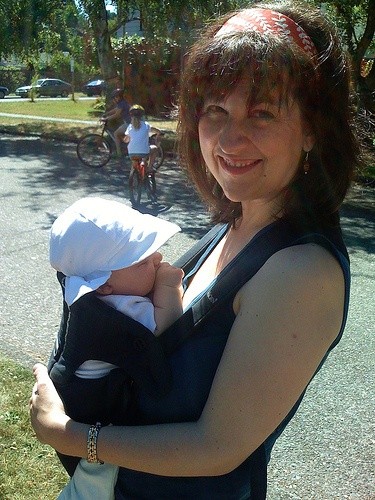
[111,88,124,96]
[129,104,144,111]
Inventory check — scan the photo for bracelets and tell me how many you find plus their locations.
[86,420,108,465]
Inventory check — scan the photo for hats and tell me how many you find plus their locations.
[49,198,181,307]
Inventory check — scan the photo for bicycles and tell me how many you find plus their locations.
[128,131,164,204]
[77,115,163,172]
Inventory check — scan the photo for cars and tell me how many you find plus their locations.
[83,80,106,98]
[0,87,10,99]
[15,79,73,98]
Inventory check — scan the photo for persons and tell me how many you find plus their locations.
[44,197,184,500]
[123,104,164,185]
[100,88,134,160]
[30,1,362,499]
